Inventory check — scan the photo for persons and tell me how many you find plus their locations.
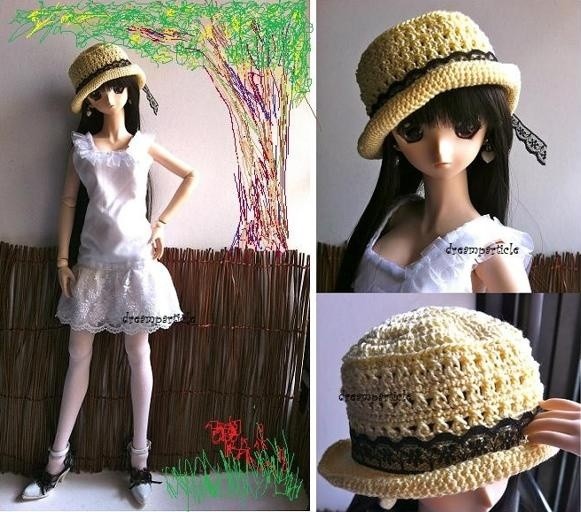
[19,39,199,508]
